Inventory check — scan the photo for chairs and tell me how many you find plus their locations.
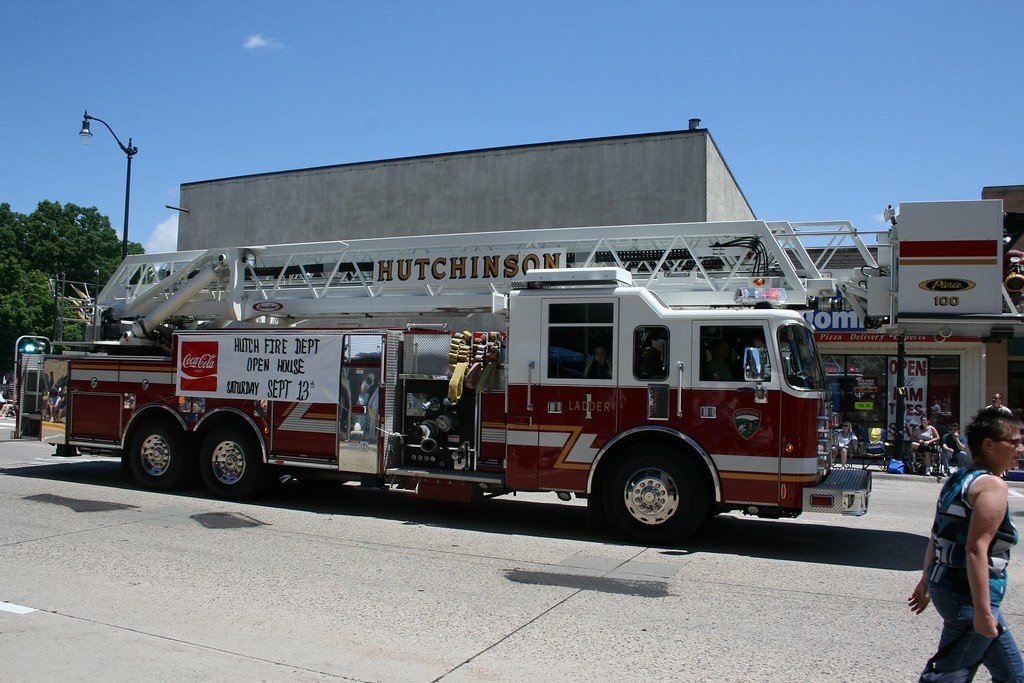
[833,429,853,468]
[909,424,944,475]
[859,427,890,472]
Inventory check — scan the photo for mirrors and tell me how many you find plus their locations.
[41,357,70,444]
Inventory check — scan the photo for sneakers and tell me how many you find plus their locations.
[918,446,931,452]
[924,467,931,476]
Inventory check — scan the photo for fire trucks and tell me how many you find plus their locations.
[65,221,879,547]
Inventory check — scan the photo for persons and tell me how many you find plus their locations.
[708,340,738,381]
[1,373,18,419]
[833,421,858,467]
[912,415,941,476]
[986,392,1013,415]
[47,383,67,423]
[941,423,966,476]
[583,348,613,379]
[909,409,1024,682]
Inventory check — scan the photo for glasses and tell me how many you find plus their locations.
[992,397,1000,400]
[1000,435,1023,447]
[950,428,957,431]
[841,425,848,427]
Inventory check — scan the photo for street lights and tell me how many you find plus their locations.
[78,110,138,262]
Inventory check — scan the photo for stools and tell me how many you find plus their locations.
[940,458,958,473]
